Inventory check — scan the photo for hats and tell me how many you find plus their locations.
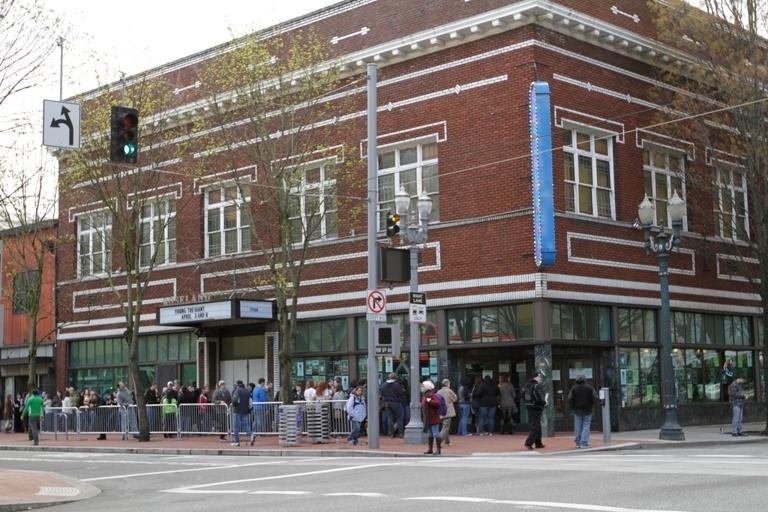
[423,381,434,391]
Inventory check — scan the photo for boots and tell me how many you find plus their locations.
[434,438,442,455]
[424,436,433,454]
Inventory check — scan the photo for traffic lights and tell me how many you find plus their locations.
[111,106,138,165]
[379,329,392,343]
[386,209,400,237]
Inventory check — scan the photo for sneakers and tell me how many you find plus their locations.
[575,440,593,449]
[457,433,494,440]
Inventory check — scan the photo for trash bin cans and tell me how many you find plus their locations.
[277,405,302,446]
[305,404,331,444]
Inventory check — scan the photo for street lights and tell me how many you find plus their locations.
[393,184,433,446]
[637,186,686,441]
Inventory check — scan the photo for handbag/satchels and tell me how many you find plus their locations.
[435,394,446,416]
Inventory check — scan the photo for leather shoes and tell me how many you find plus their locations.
[524,441,546,451]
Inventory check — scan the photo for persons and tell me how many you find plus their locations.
[456,374,516,438]
[521,372,549,449]
[292,376,367,445]
[420,377,457,455]
[566,374,601,449]
[21,388,45,445]
[145,378,280,446]
[41,380,138,440]
[4,392,29,434]
[726,374,749,436]
[378,372,411,439]
